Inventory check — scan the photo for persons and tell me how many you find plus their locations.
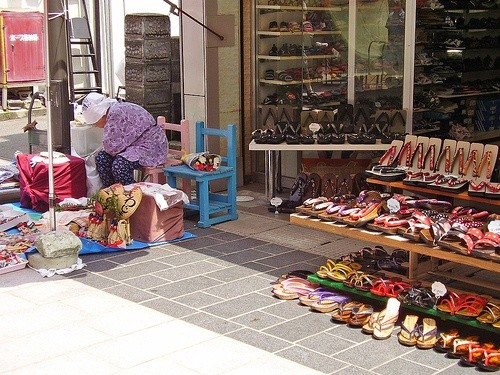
[82,91,168,186]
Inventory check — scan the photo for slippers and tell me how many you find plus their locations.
[251,0,500,371]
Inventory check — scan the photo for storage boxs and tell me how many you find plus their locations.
[0,202,30,274]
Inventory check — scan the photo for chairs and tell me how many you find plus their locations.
[162,120,238,228]
[136,116,190,204]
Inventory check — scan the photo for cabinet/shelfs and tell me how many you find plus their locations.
[249,0,500,171]
[290,174,500,343]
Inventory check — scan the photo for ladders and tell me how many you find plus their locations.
[65,0,103,103]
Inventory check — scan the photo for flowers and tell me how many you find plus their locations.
[180,149,222,172]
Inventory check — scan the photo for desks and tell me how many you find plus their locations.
[249,132,404,213]
